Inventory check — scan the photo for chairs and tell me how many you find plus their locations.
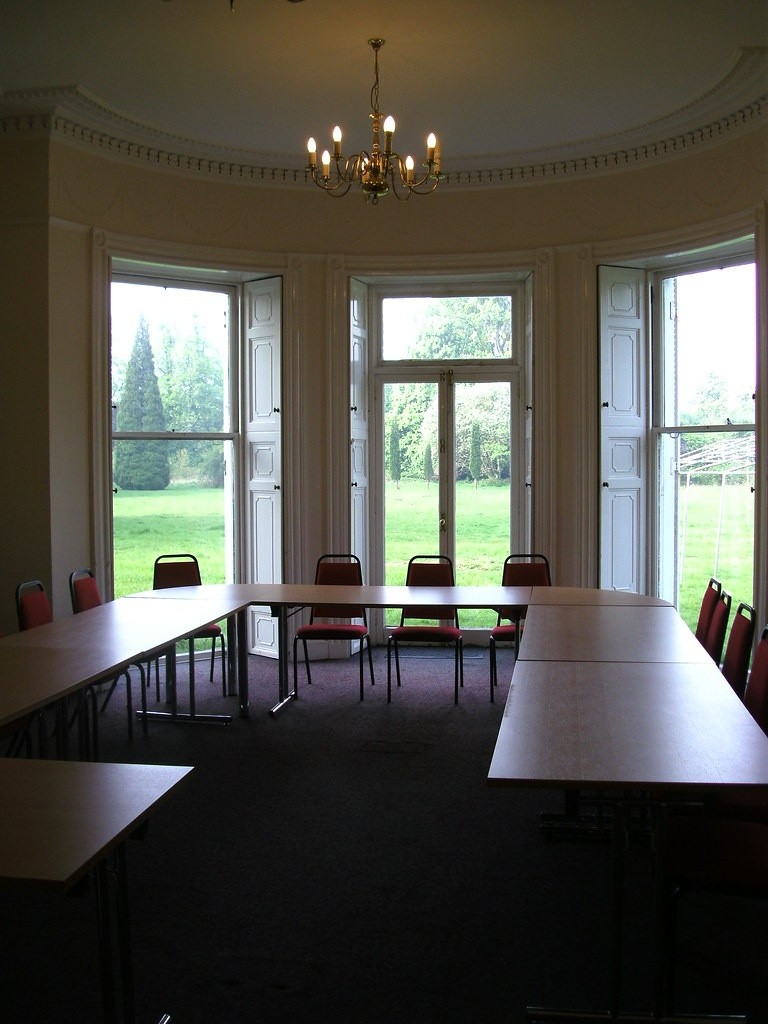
[148,554,226,703]
[69,568,178,736]
[490,553,551,703]
[293,554,375,701]
[694,578,767,739]
[15,579,134,763]
[387,556,463,704]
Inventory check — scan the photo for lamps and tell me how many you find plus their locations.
[306,48,441,206]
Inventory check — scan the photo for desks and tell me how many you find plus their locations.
[0,582,768,1024]
